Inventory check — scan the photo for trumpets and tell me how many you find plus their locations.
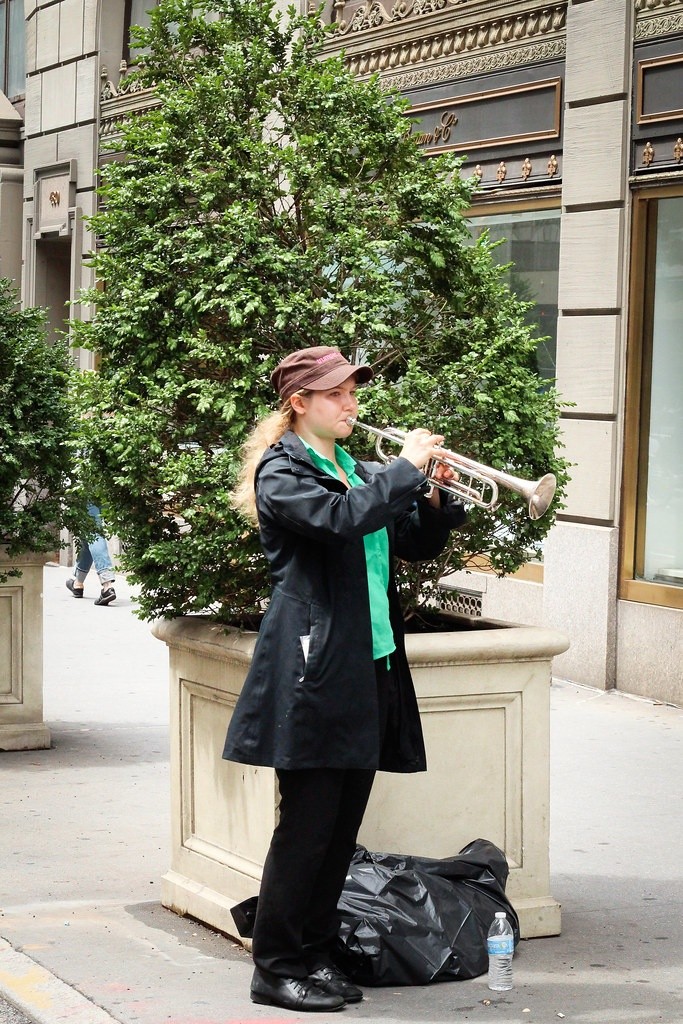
[345,416,555,520]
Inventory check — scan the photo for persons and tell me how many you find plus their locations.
[63,413,117,605]
[222,345,460,1013]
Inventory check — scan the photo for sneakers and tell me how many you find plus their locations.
[65,577,84,599]
[94,586,116,606]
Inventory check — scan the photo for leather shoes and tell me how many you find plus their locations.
[247,975,348,1014]
[309,968,364,1004]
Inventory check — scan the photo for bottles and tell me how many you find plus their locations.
[487,912,515,991]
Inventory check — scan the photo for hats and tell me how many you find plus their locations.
[270,345,376,406]
